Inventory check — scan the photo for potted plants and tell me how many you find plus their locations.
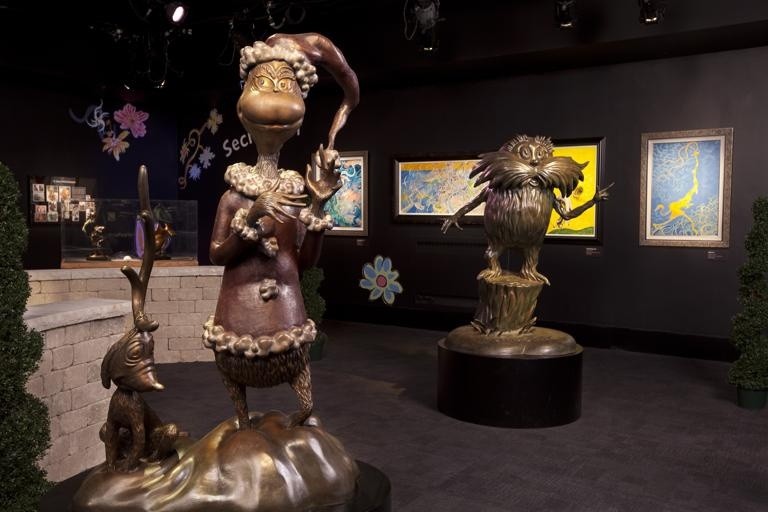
[726,195,768,412]
[301,267,329,363]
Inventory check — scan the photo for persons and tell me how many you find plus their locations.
[203,38,345,431]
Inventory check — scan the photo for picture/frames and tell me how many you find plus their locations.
[542,137,608,246]
[635,127,736,250]
[310,150,370,239]
[390,151,485,227]
